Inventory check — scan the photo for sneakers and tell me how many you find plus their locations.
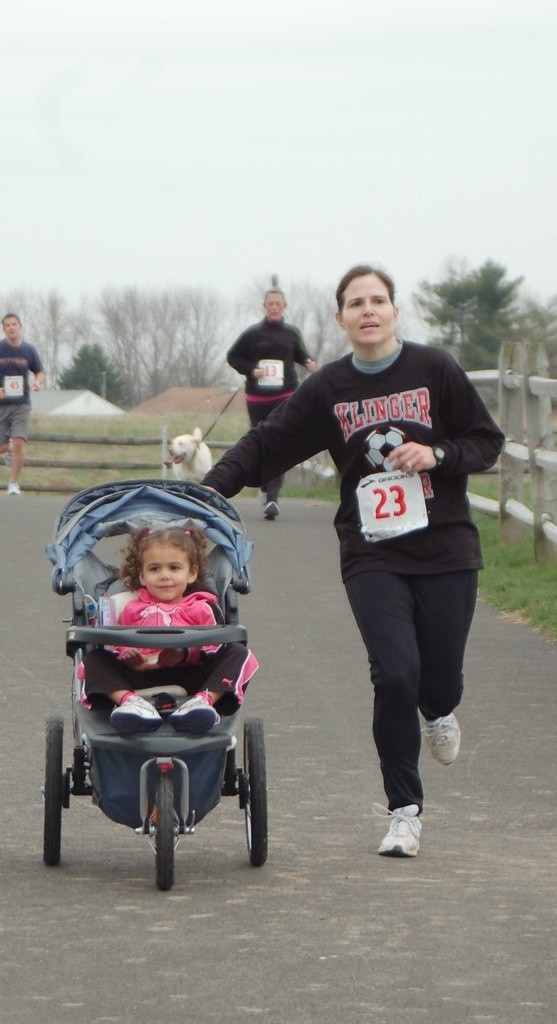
[109,695,163,736]
[165,685,222,734]
[371,801,422,858]
[419,710,461,765]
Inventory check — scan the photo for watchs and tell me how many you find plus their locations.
[432,445,445,465]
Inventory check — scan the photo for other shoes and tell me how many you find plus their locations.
[264,500,281,523]
[6,481,22,496]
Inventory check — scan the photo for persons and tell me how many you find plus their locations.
[83,527,259,734]
[201,264,504,858]
[0,314,43,494]
[226,286,314,520]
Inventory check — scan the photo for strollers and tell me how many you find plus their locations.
[41,479,269,891]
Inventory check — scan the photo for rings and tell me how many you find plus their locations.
[405,461,412,468]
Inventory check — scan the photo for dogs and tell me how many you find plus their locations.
[169,427,212,485]
